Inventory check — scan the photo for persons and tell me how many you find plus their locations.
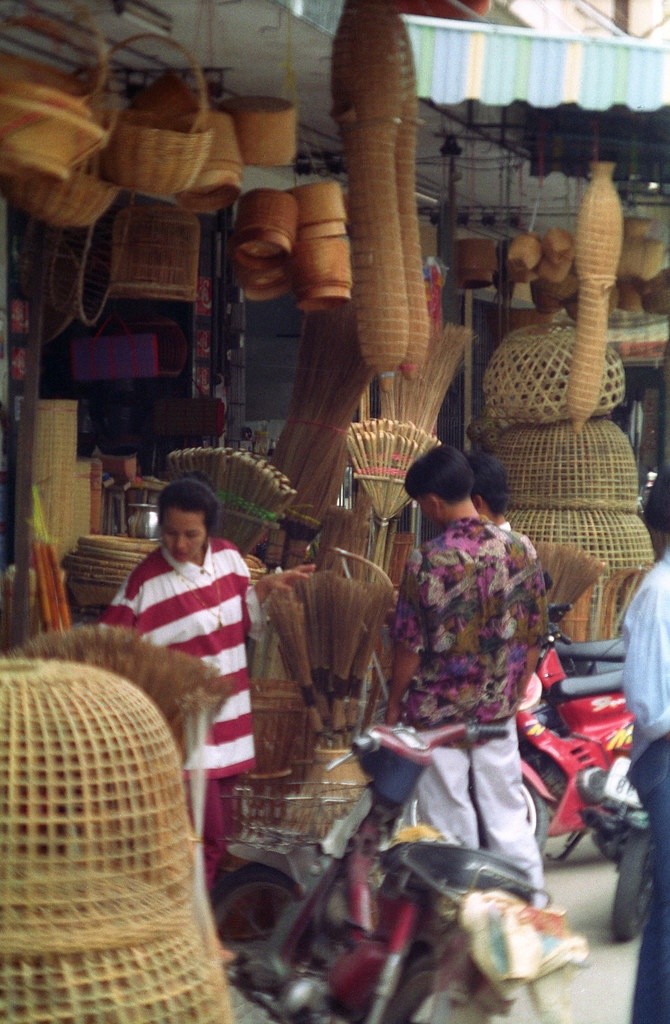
[100,481,313,968]
[621,457,670,1024]
[384,446,550,910]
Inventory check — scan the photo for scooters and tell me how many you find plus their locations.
[207,720,552,1024]
[466,570,670,943]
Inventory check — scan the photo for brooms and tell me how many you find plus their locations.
[345,323,481,588]
[528,538,653,642]
[167,446,297,558]
[1,622,227,842]
[259,572,393,751]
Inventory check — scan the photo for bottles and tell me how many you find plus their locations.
[128,503,161,538]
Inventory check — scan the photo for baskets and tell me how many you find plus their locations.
[0,0,669,645]
[0,654,234,1024]
[235,682,370,847]
